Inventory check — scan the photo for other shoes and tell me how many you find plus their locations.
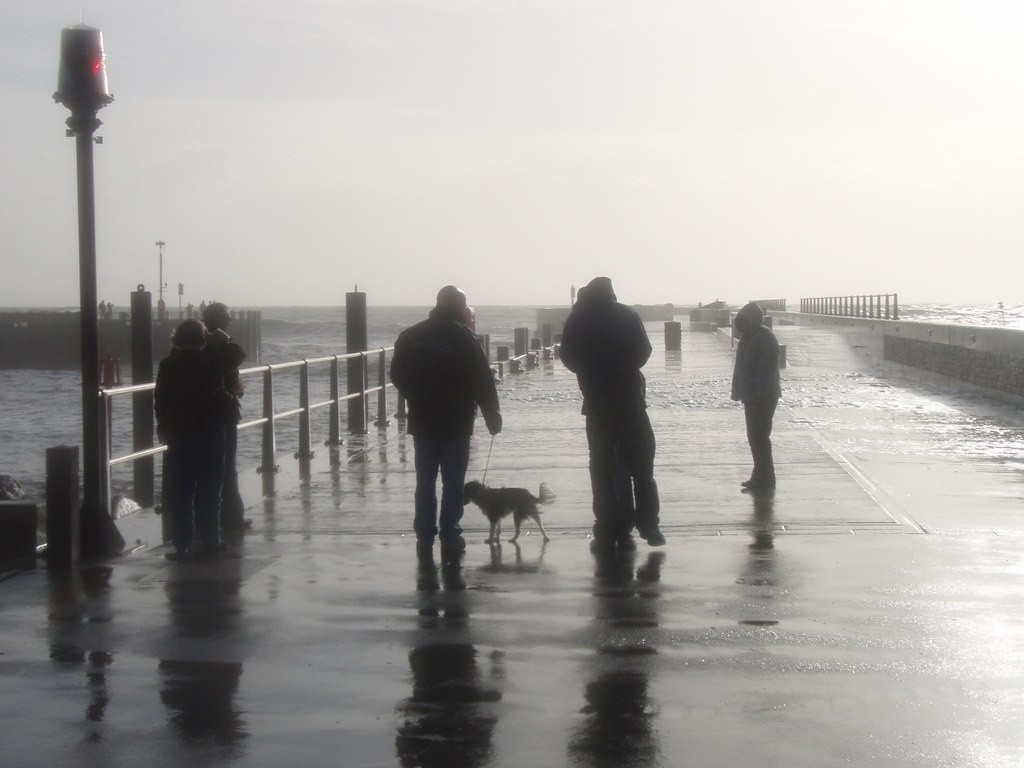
[165,549,191,560]
[640,523,666,546]
[742,480,776,490]
[223,517,253,531]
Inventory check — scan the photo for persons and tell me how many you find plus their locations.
[158,299,165,320]
[389,285,503,541]
[107,303,114,319]
[199,300,206,317]
[99,300,106,319]
[730,301,782,487]
[209,300,215,304]
[152,303,253,554]
[186,304,193,317]
[560,277,666,551]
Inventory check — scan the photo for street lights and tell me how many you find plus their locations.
[51,19,128,560]
[154,238,166,320]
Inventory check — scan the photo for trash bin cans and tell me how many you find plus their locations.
[664,321,682,351]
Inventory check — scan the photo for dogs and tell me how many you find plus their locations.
[463,480,556,545]
